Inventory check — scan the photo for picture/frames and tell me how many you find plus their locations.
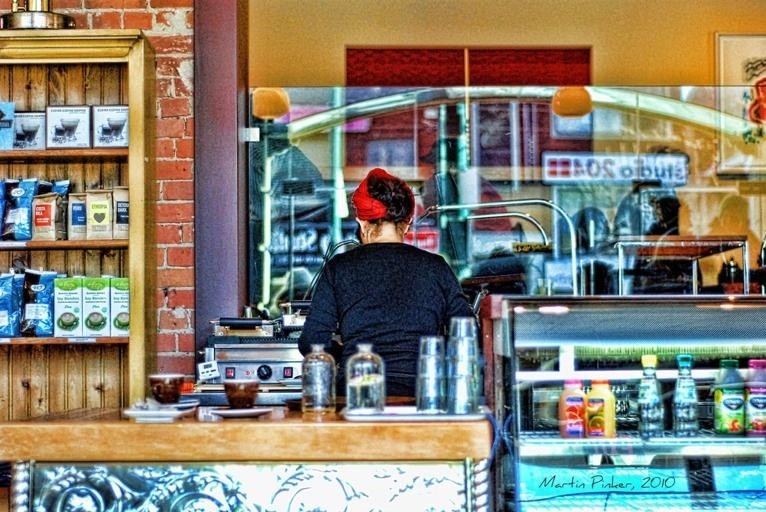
[712,33,765,174]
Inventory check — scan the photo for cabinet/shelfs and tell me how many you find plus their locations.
[470,293,765,512]
[1,30,159,413]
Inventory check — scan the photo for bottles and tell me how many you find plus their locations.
[636,354,665,437]
[673,355,698,439]
[346,343,385,411]
[300,343,338,416]
[744,360,766,440]
[714,357,745,434]
[555,379,621,437]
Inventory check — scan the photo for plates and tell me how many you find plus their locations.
[210,406,273,419]
[123,406,197,424]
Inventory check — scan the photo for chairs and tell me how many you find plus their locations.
[431,169,579,296]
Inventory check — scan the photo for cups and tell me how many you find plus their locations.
[414,315,483,414]
[224,378,261,408]
[147,373,185,403]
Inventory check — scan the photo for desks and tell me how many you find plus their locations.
[580,232,752,295]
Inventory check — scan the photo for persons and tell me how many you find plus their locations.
[566,206,616,300]
[417,134,515,232]
[296,169,481,399]
[245,120,332,226]
[703,193,765,297]
[631,197,702,292]
[613,144,693,289]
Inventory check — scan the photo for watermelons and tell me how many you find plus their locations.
[565,405,585,420]
